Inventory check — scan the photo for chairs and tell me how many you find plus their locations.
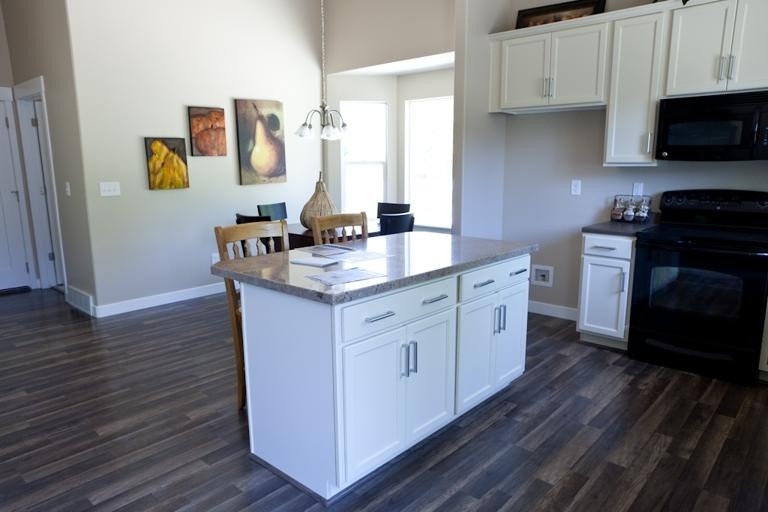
[232,211,279,260]
[256,200,289,222]
[379,211,416,235]
[376,201,412,219]
[310,209,368,251]
[211,215,292,413]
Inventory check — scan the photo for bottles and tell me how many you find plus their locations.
[611,197,651,222]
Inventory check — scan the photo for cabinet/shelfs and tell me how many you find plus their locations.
[236,251,458,508]
[453,245,528,418]
[485,19,610,117]
[574,215,658,352]
[663,0,768,98]
[600,2,666,170]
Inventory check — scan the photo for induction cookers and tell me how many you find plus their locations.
[634,220,768,241]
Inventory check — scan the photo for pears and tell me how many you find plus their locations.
[250,116,284,178]
[300,181,335,232]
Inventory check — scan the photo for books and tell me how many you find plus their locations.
[290,255,338,268]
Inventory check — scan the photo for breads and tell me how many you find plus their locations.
[190,110,226,156]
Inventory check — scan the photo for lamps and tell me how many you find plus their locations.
[294,0,348,144]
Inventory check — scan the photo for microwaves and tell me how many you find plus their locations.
[650,90,768,162]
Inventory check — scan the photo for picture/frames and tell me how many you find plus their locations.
[511,1,608,31]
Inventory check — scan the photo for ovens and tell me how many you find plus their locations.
[626,244,767,386]
